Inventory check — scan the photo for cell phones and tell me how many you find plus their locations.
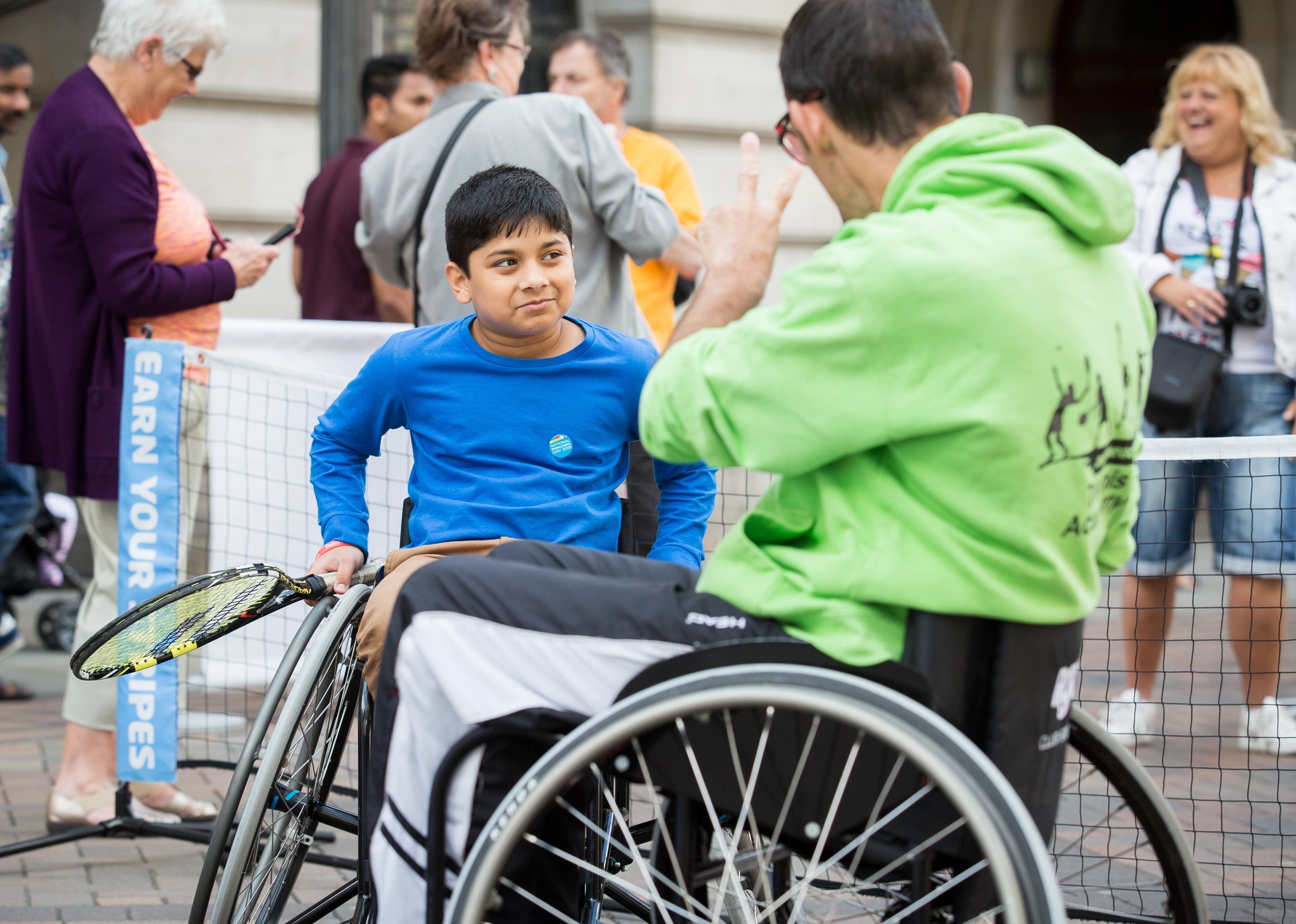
[264,223,297,246]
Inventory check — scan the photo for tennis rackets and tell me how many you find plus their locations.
[68,559,386,682]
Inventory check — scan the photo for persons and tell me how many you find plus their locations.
[290,0,705,352]
[1,1,281,840]
[302,168,717,609]
[1,43,38,700]
[1100,42,1296,758]
[363,0,1161,924]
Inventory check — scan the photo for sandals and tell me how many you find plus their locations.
[46,784,183,838]
[129,777,220,822]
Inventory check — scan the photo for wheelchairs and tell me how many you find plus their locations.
[195,583,1210,924]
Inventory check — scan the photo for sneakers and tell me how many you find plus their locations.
[1097,687,1155,749]
[1235,695,1296,753]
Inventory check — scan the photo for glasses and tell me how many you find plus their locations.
[502,42,532,61]
[176,53,202,80]
[774,90,823,166]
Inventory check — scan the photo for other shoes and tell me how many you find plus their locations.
[0,679,35,702]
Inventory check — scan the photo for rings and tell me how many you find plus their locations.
[1185,300,1195,306]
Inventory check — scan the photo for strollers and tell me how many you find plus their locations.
[6,493,89,652]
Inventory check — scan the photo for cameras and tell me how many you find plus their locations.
[1215,282,1267,327]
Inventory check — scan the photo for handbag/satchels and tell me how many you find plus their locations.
[1145,333,1221,439]
